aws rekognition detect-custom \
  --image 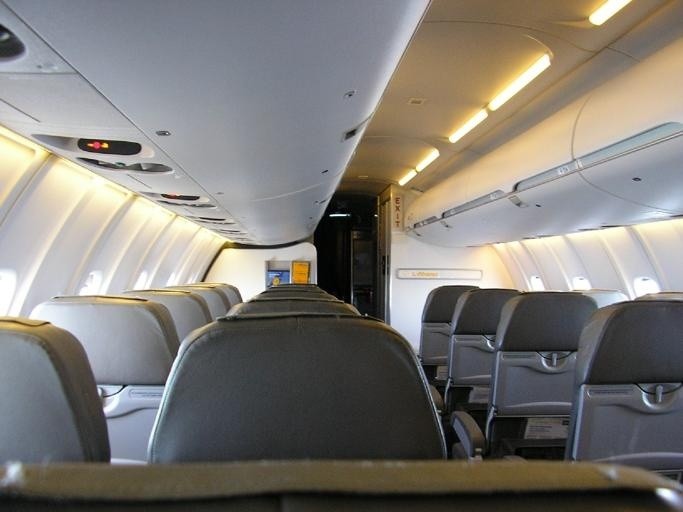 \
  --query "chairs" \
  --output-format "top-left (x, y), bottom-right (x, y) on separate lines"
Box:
top-left (28, 285), bottom-right (361, 464)
top-left (567, 291), bottom-right (683, 480)
top-left (1, 313), bottom-right (112, 462)
top-left (419, 283), bottom-right (629, 460)
top-left (146, 313), bottom-right (448, 460)
top-left (1, 459), bottom-right (683, 512)
